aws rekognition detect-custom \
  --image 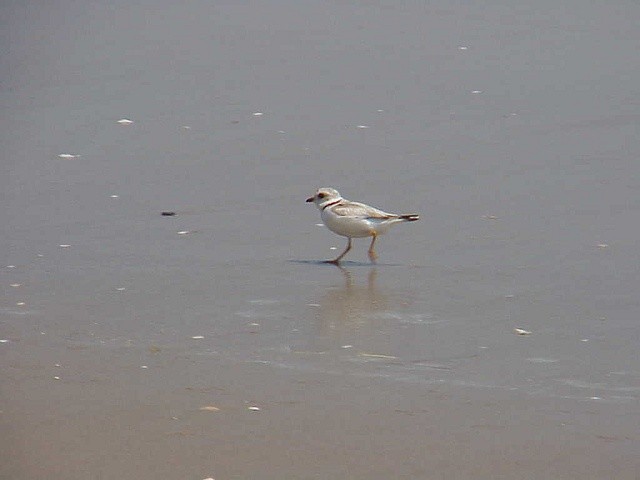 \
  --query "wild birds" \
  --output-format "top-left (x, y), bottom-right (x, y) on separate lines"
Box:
top-left (306, 184), bottom-right (422, 269)
